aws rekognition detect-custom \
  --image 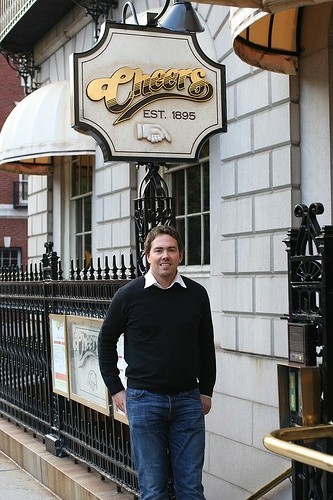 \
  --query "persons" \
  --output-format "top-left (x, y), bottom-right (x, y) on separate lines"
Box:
top-left (98, 225), bottom-right (216, 499)
